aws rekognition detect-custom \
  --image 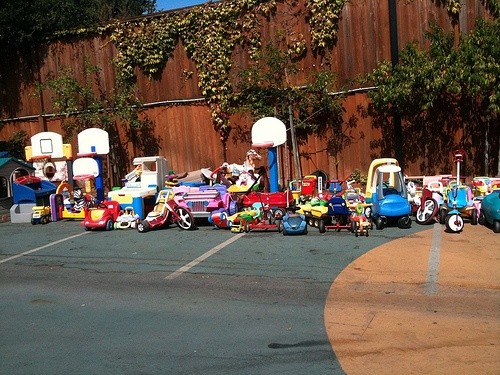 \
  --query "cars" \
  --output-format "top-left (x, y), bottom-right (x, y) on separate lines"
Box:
top-left (12, 141), bottom-right (499, 240)
